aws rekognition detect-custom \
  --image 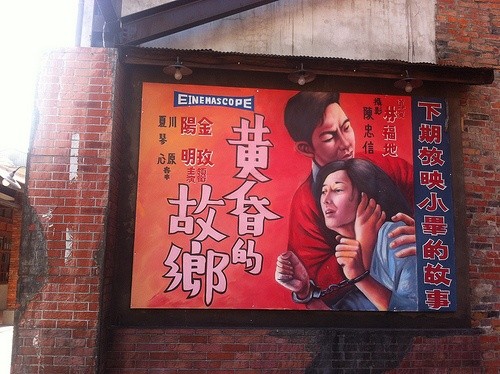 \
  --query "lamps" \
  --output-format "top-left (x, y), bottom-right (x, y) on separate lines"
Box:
top-left (0, 165), bottom-right (27, 191)
top-left (288, 59), bottom-right (317, 86)
top-left (163, 54), bottom-right (193, 82)
top-left (394, 68), bottom-right (422, 94)
